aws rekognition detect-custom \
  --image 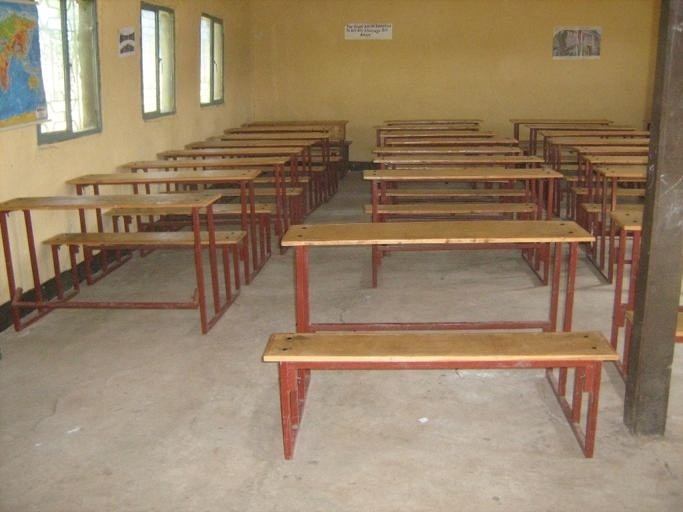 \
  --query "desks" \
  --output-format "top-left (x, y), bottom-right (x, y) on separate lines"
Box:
top-left (1, 121), bottom-right (262, 336)
top-left (262, 112), bottom-right (681, 464)
